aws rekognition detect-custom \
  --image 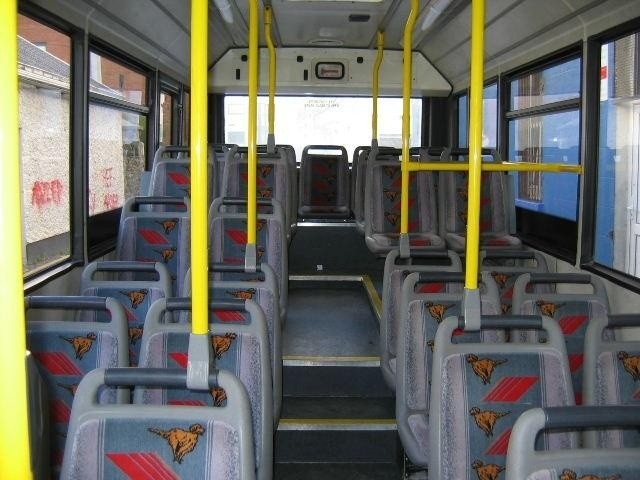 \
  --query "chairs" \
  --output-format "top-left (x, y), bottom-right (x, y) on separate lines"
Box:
top-left (296, 144), bottom-right (356, 221)
top-left (22, 144), bottom-right (298, 480)
top-left (350, 142), bottom-right (640, 479)
